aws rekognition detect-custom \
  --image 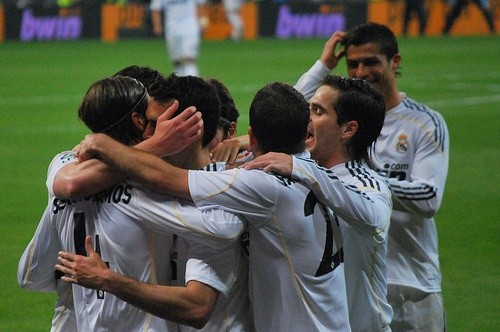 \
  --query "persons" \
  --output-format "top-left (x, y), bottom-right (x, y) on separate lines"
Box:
top-left (0, 0), bottom-right (497, 332)
top-left (294, 22), bottom-right (449, 332)
